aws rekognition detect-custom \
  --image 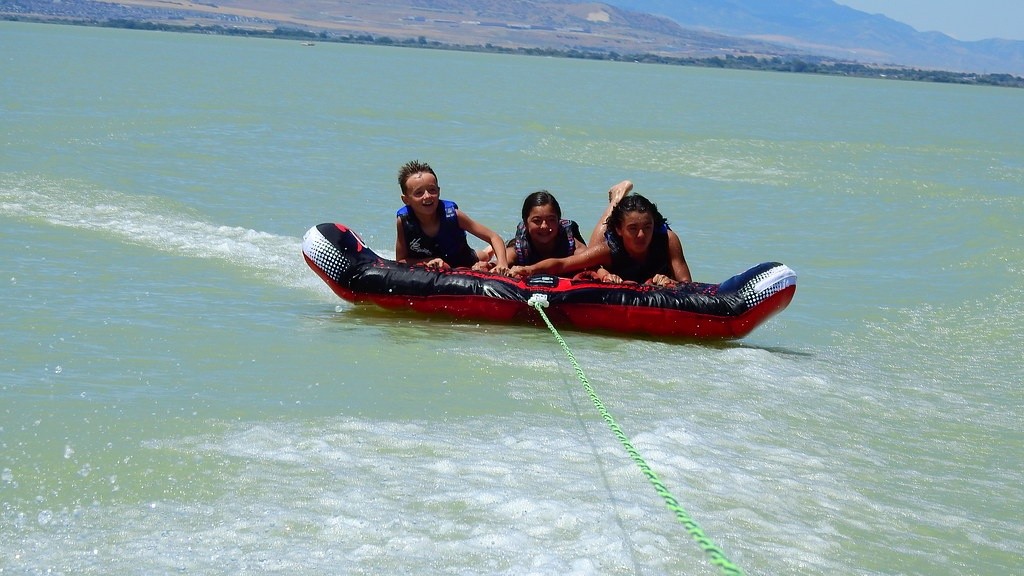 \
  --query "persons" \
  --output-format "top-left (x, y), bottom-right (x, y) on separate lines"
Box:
top-left (395, 160), bottom-right (512, 276)
top-left (509, 179), bottom-right (692, 286)
top-left (471, 190), bottom-right (625, 284)
